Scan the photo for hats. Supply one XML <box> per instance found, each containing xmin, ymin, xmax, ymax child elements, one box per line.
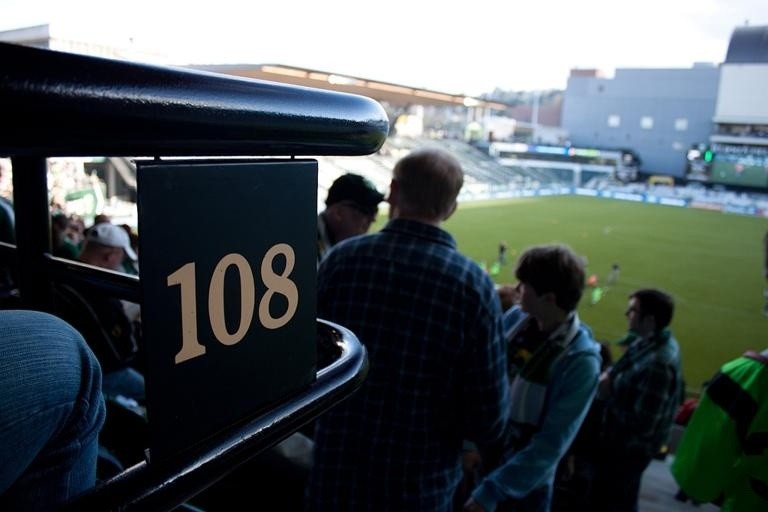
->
<box><xmin>327</xmin><ymin>173</ymin><xmax>383</xmax><ymax>209</ymax></box>
<box><xmin>87</xmin><ymin>223</ymin><xmax>139</xmax><ymax>261</ymax></box>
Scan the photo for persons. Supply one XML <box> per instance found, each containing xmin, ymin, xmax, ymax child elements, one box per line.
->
<box><xmin>316</xmin><ymin>150</ymin><xmax>511</xmax><ymax>512</ymax></box>
<box><xmin>462</xmin><ymin>242</ymin><xmax>681</xmax><ymax>512</ymax></box>
<box><xmin>1</xmin><ymin>310</ymin><xmax>106</xmax><ymax>511</ymax></box>
<box><xmin>669</xmin><ymin>345</ymin><xmax>766</xmax><ymax>511</ymax></box>
<box><xmin>1</xmin><ymin>190</ymin><xmax>146</xmax><ymax>469</ymax></box>
